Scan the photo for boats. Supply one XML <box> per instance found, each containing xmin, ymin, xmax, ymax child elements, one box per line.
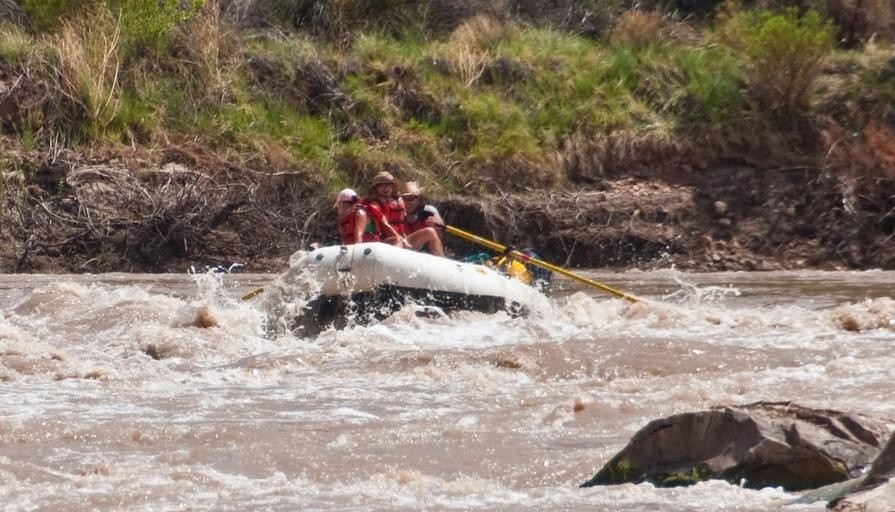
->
<box><xmin>249</xmin><ymin>240</ymin><xmax>555</xmax><ymax>336</ymax></box>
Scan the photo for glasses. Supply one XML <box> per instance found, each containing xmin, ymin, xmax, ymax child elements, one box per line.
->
<box><xmin>379</xmin><ymin>183</ymin><xmax>393</xmax><ymax>187</ymax></box>
<box><xmin>403</xmin><ymin>197</ymin><xmax>414</xmax><ymax>202</ymax></box>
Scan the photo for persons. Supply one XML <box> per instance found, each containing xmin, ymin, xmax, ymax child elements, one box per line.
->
<box><xmin>330</xmin><ymin>187</ymin><xmax>377</xmax><ymax>244</ymax></box>
<box><xmin>397</xmin><ymin>179</ymin><xmax>446</xmax><ymax>255</ymax></box>
<box><xmin>364</xmin><ymin>171</ymin><xmax>446</xmax><ymax>257</ymax></box>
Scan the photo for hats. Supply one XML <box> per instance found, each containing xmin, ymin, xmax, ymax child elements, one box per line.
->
<box><xmin>332</xmin><ymin>188</ymin><xmax>358</xmax><ymax>209</ymax></box>
<box><xmin>368</xmin><ymin>171</ymin><xmax>395</xmax><ymax>189</ymax></box>
<box><xmin>400</xmin><ymin>181</ymin><xmax>420</xmax><ymax>196</ymax></box>
